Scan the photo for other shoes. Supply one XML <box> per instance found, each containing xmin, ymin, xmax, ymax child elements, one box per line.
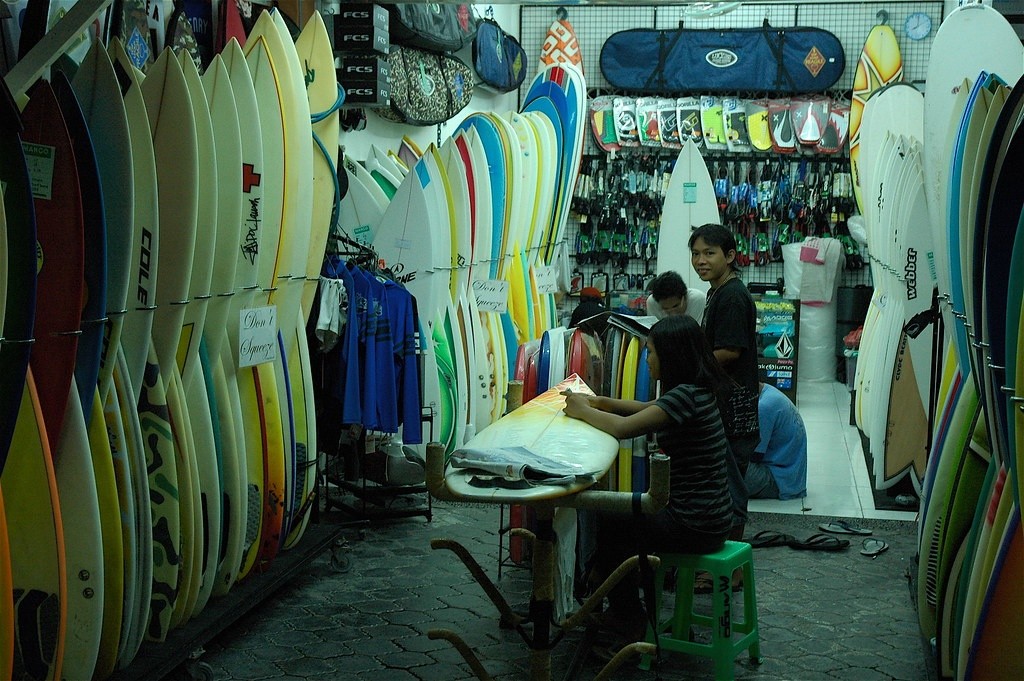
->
<box><xmin>596</xmin><ymin>602</ymin><xmax>647</xmax><ymax>640</ymax></box>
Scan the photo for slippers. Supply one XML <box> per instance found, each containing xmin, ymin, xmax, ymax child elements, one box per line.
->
<box><xmin>741</xmin><ymin>530</ymin><xmax>795</xmax><ymax>547</ymax></box>
<box><xmin>788</xmin><ymin>533</ymin><xmax>849</xmax><ymax>550</ymax></box>
<box><xmin>860</xmin><ymin>538</ymin><xmax>889</xmax><ymax>556</ymax></box>
<box><xmin>819</xmin><ymin>520</ymin><xmax>873</xmax><ymax>536</ymax></box>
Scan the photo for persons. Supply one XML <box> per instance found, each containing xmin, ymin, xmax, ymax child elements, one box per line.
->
<box><xmin>568</xmin><ymin>287</ymin><xmax>608</xmax><ymax>329</ymax></box>
<box><xmin>563</xmin><ymin>316</ymin><xmax>734</xmax><ymax>642</ymax></box>
<box><xmin>646</xmin><ymin>270</ymin><xmax>706</xmax><ymax>326</ymax></box>
<box><xmin>688</xmin><ymin>224</ymin><xmax>758</xmax><ymax>594</ymax></box>
<box><xmin>745</xmin><ymin>382</ymin><xmax>807</xmax><ymax>501</ymax></box>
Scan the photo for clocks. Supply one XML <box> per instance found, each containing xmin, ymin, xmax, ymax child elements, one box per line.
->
<box><xmin>903</xmin><ymin>12</ymin><xmax>932</xmax><ymax>41</ymax></box>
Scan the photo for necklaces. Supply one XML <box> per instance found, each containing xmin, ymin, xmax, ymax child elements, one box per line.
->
<box><xmin>704</xmin><ymin>271</ymin><xmax>734</xmax><ymax>311</ymax></box>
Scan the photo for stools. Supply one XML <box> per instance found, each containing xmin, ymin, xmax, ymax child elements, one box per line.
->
<box><xmin>638</xmin><ymin>541</ymin><xmax>761</xmax><ymax>681</ymax></box>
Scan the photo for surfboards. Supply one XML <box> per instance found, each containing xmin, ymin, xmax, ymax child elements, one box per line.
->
<box><xmin>0</xmin><ymin>4</ymin><xmax>1024</xmax><ymax>680</ymax></box>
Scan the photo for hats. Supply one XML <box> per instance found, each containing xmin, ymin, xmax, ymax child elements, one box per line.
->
<box><xmin>580</xmin><ymin>287</ymin><xmax>602</xmax><ymax>302</ymax></box>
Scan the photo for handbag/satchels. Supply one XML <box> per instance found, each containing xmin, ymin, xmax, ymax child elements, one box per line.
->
<box><xmin>452</xmin><ymin>6</ymin><xmax>527</xmax><ymax>94</ymax></box>
<box><xmin>354</xmin><ymin>44</ymin><xmax>473</xmax><ymax>126</ymax></box>
<box><xmin>599</xmin><ymin>20</ymin><xmax>845</xmax><ymax>89</ymax></box>
<box><xmin>389</xmin><ymin>2</ymin><xmax>478</xmax><ymax>52</ymax></box>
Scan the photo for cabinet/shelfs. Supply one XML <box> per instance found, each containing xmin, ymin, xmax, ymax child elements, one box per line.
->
<box><xmin>325</xmin><ymin>405</ymin><xmax>435</xmax><ymax>523</ymax></box>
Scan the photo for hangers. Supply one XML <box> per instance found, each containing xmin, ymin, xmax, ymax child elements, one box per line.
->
<box><xmin>324</xmin><ymin>238</ymin><xmax>412</xmax><ymax>280</ymax></box>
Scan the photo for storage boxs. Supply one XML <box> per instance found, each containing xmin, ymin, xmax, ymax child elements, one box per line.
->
<box><xmin>333</xmin><ymin>2</ymin><xmax>392</xmax><ymax>106</ymax></box>
